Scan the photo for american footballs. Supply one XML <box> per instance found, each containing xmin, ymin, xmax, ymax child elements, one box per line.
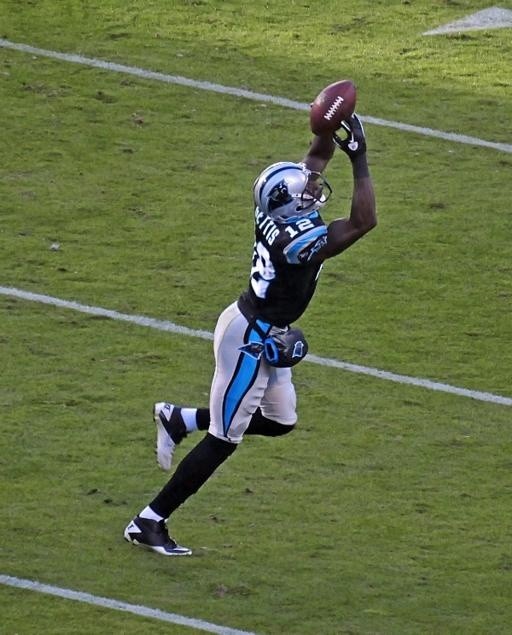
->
<box><xmin>310</xmin><ymin>80</ymin><xmax>356</xmax><ymax>133</ymax></box>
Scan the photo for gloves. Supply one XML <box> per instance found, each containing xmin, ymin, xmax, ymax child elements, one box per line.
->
<box><xmin>329</xmin><ymin>112</ymin><xmax>370</xmax><ymax>164</ymax></box>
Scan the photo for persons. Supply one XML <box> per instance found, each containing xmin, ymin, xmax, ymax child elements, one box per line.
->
<box><xmin>123</xmin><ymin>107</ymin><xmax>380</xmax><ymax>559</ymax></box>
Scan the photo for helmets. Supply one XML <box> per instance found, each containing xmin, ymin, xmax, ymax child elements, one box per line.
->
<box><xmin>250</xmin><ymin>159</ymin><xmax>334</xmax><ymax>224</ymax></box>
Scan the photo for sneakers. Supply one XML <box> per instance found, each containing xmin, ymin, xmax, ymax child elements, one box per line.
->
<box><xmin>121</xmin><ymin>513</ymin><xmax>194</xmax><ymax>558</ymax></box>
<box><xmin>151</xmin><ymin>400</ymin><xmax>192</xmax><ymax>472</ymax></box>
